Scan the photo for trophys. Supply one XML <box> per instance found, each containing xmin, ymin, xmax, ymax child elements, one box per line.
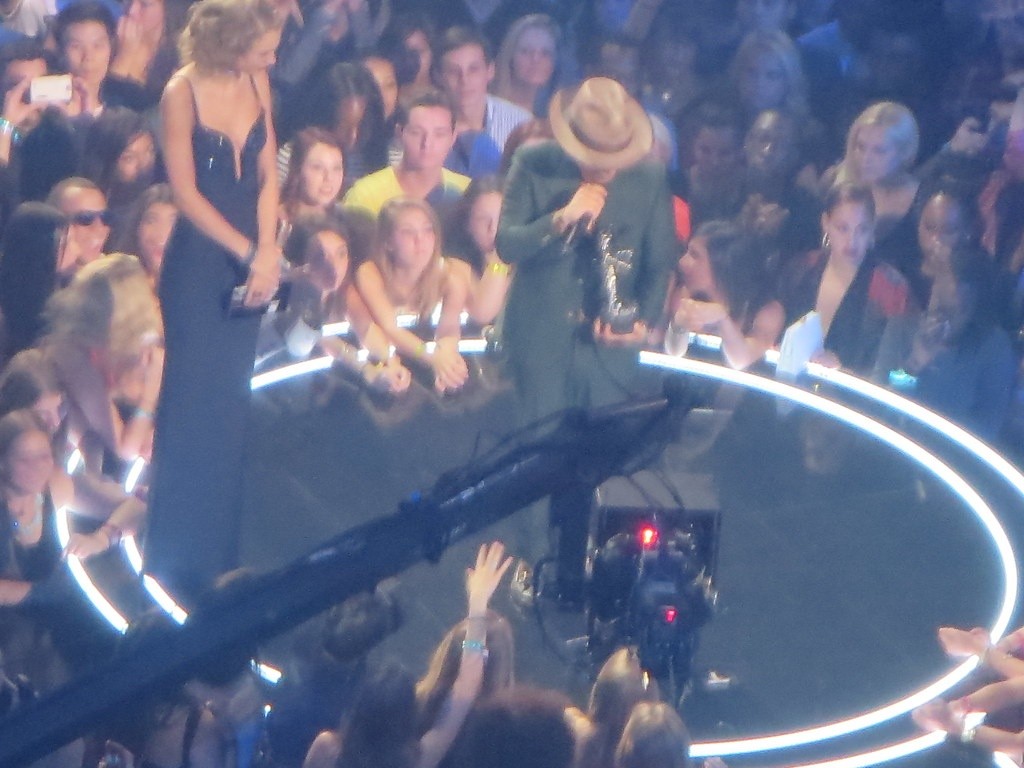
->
<box><xmin>594</xmin><ymin>226</ymin><xmax>640</xmax><ymax>335</ymax></box>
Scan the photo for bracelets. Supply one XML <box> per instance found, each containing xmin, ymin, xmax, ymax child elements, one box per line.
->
<box><xmin>461</xmin><ymin>640</ymin><xmax>483</xmax><ymax>650</ymax></box>
<box><xmin>963</xmin><ymin>697</ymin><xmax>971</xmax><ymax>714</ymax></box>
<box><xmin>12</xmin><ymin>129</ymin><xmax>23</xmax><ymax>147</ymax></box>
<box><xmin>0</xmin><ymin>117</ymin><xmax>13</xmax><ymax>134</ymax></box>
<box><xmin>961</xmin><ymin>727</ymin><xmax>975</xmax><ymax>743</ymax></box>
<box><xmin>978</xmin><ymin>642</ymin><xmax>995</xmax><ymax>664</ymax></box>
<box><xmin>133</xmin><ymin>409</ymin><xmax>157</xmax><ymax>418</ymax></box>
<box><xmin>101</xmin><ymin>524</ymin><xmax>120</xmax><ymax>548</ymax></box>
<box><xmin>487</xmin><ymin>264</ymin><xmax>508</xmax><ymax>274</ymax></box>
<box><xmin>414</xmin><ymin>342</ymin><xmax>427</xmax><ymax>359</ymax></box>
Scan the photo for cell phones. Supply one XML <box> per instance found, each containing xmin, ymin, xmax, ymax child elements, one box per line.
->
<box><xmin>31</xmin><ymin>74</ymin><xmax>73</xmax><ymax>103</ymax></box>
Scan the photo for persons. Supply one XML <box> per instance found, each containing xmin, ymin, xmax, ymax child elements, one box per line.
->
<box><xmin>0</xmin><ymin>0</ymin><xmax>1024</xmax><ymax>768</ymax></box>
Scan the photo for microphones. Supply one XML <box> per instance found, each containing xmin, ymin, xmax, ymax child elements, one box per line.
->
<box><xmin>559</xmin><ymin>222</ymin><xmax>578</xmax><ymax>257</ymax></box>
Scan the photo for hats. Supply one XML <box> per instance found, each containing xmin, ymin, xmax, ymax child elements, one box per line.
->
<box><xmin>550</xmin><ymin>75</ymin><xmax>656</xmax><ymax>171</ymax></box>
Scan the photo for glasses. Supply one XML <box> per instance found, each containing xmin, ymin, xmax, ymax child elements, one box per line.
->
<box><xmin>68</xmin><ymin>209</ymin><xmax>113</xmax><ymax>226</ymax></box>
